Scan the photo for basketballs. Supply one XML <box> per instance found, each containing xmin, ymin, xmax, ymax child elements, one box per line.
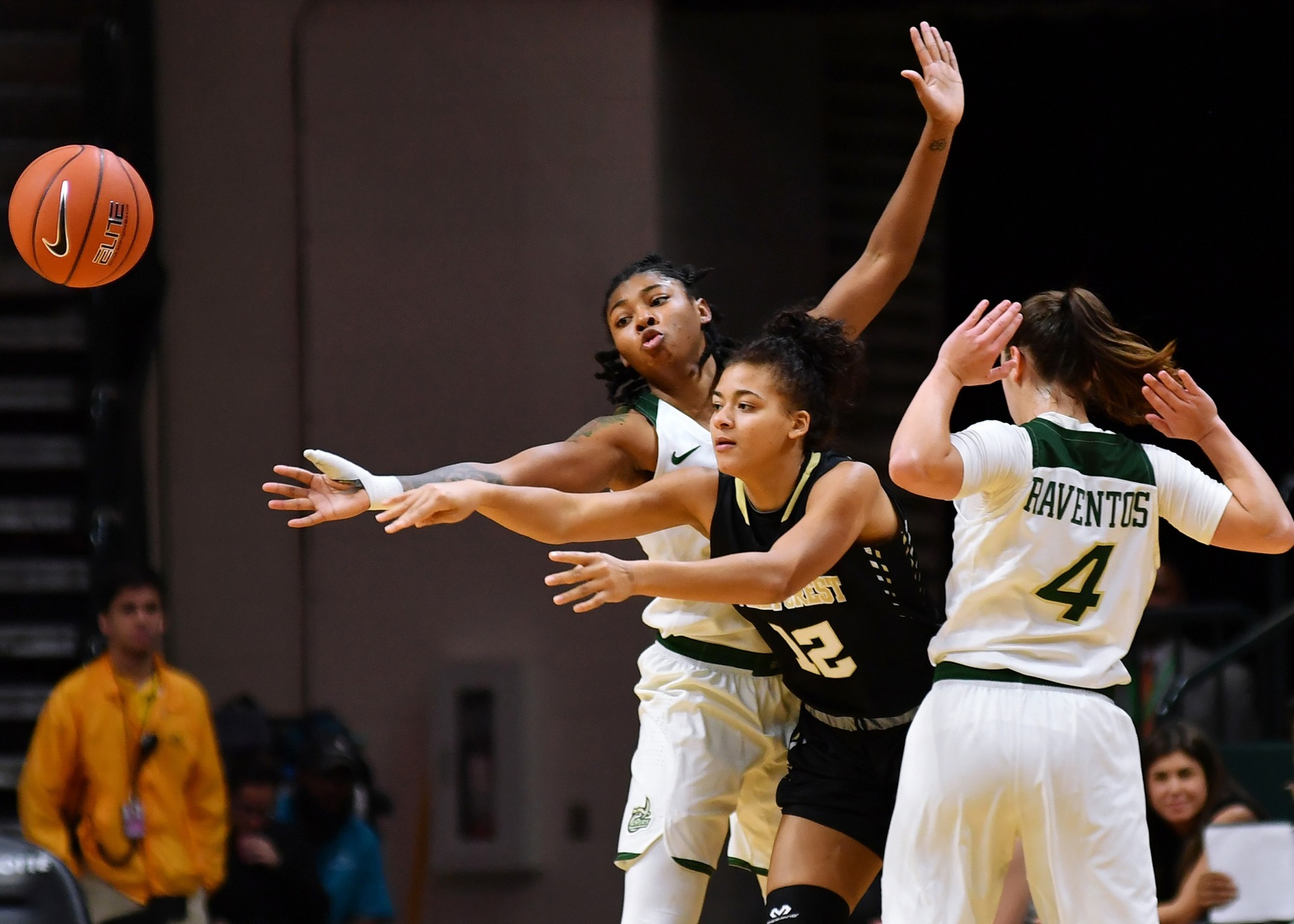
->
<box><xmin>7</xmin><ymin>145</ymin><xmax>154</xmax><ymax>289</ymax></box>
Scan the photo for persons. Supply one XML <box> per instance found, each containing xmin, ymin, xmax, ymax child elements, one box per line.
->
<box><xmin>260</xmin><ymin>19</ymin><xmax>965</xmax><ymax>924</ymax></box>
<box><xmin>18</xmin><ymin>562</ymin><xmax>230</xmax><ymax>924</ymax></box>
<box><xmin>880</xmin><ymin>286</ymin><xmax>1294</xmax><ymax>924</ymax></box>
<box><xmin>373</xmin><ymin>307</ymin><xmax>934</xmax><ymax>924</ymax></box>
<box><xmin>211</xmin><ymin>693</ymin><xmax>391</xmax><ymax>924</ymax></box>
<box><xmin>1145</xmin><ymin>718</ymin><xmax>1253</xmax><ymax>924</ymax></box>
<box><xmin>1119</xmin><ymin>557</ymin><xmax>1255</xmax><ymax>745</ymax></box>
